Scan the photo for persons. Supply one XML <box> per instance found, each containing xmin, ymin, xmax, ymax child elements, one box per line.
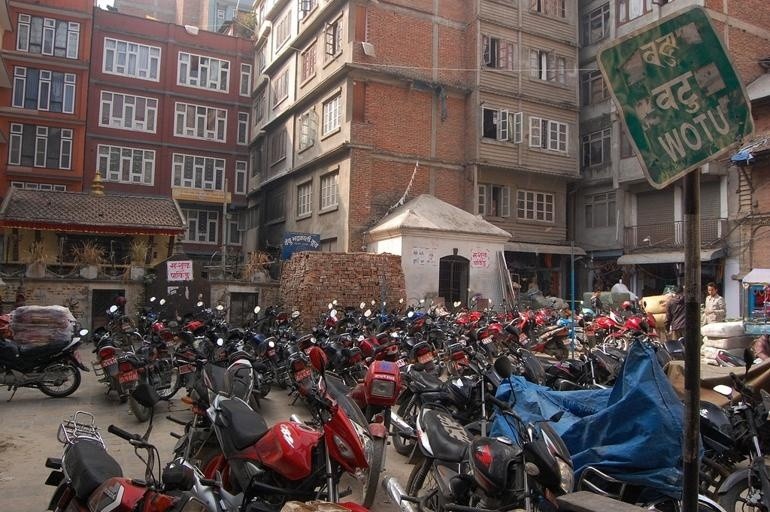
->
<box><xmin>664</xmin><ymin>283</ymin><xmax>686</xmax><ymax>340</ymax></box>
<box><xmin>590</xmin><ymin>288</ymin><xmax>606</xmax><ymax>315</ymax></box>
<box><xmin>751</xmin><ymin>334</ymin><xmax>770</xmax><ymax>360</ymax></box>
<box><xmin>699</xmin><ymin>281</ymin><xmax>726</xmax><ymax>327</ymax></box>
<box><xmin>528</xmin><ymin>276</ymin><xmax>539</xmax><ymax>290</ymax></box>
